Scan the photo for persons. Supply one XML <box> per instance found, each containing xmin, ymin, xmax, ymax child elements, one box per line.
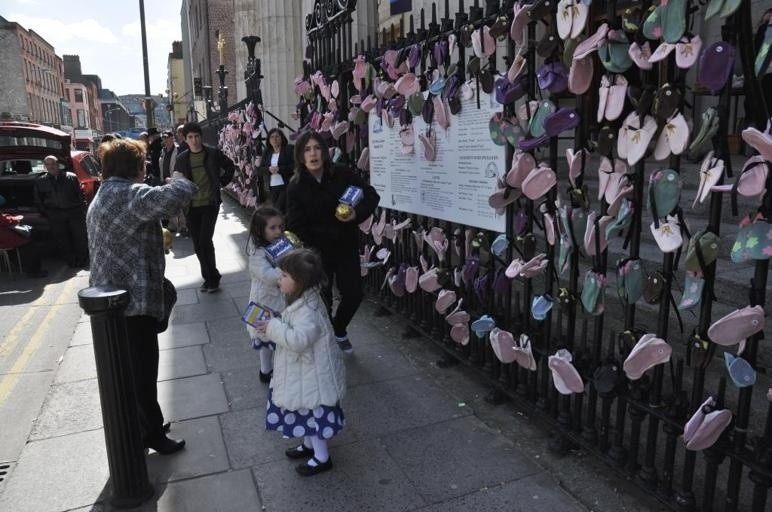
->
<box><xmin>246</xmin><ymin>206</ymin><xmax>284</xmax><ymax>383</ymax></box>
<box><xmin>85</xmin><ymin>137</ymin><xmax>199</xmax><ymax>455</ymax></box>
<box><xmin>33</xmin><ymin>155</ymin><xmax>88</xmax><ymax>269</ymax></box>
<box><xmin>257</xmin><ymin>128</ymin><xmax>296</xmax><ymax>210</ymax></box>
<box><xmin>101</xmin><ymin>121</ymin><xmax>234</xmax><ymax>293</ymax></box>
<box><xmin>1</xmin><ymin>195</ymin><xmax>49</xmax><ymax>278</ymax></box>
<box><xmin>283</xmin><ymin>129</ymin><xmax>381</xmax><ymax>353</ymax></box>
<box><xmin>254</xmin><ymin>247</ymin><xmax>347</xmax><ymax>477</ymax></box>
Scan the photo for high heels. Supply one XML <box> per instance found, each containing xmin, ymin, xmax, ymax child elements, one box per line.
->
<box><xmin>143</xmin><ymin>431</ymin><xmax>185</xmax><ymax>456</ymax></box>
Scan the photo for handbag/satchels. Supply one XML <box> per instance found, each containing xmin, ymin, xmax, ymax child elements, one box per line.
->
<box><xmin>9</xmin><ymin>220</ymin><xmax>33</xmax><ymax>238</ymax></box>
<box><xmin>136</xmin><ymin>273</ymin><xmax>178</xmax><ymax>334</ymax></box>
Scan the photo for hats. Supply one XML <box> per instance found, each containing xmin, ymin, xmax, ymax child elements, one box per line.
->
<box><xmin>162</xmin><ymin>132</ymin><xmax>173</xmax><ymax>138</ymax></box>
<box><xmin>145</xmin><ymin>127</ymin><xmax>160</xmax><ymax>138</ymax></box>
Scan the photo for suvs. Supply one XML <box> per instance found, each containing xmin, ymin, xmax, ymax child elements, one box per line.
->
<box><xmin>0</xmin><ymin>121</ymin><xmax>102</xmax><ymax>260</ymax></box>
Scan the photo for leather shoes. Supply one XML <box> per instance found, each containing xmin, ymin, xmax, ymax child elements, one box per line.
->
<box><xmin>260</xmin><ymin>367</ymin><xmax>272</xmax><ymax>384</ymax></box>
<box><xmin>296</xmin><ymin>455</ymin><xmax>333</xmax><ymax>477</ymax></box>
<box><xmin>285</xmin><ymin>443</ymin><xmax>314</xmax><ymax>458</ymax></box>
<box><xmin>162</xmin><ymin>421</ymin><xmax>171</xmax><ymax>431</ymax></box>
<box><xmin>27</xmin><ymin>269</ymin><xmax>48</xmax><ymax>277</ymax></box>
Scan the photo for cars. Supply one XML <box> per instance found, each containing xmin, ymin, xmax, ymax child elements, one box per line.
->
<box><xmin>117</xmin><ymin>126</ymin><xmax>148</xmax><ymax>140</ymax></box>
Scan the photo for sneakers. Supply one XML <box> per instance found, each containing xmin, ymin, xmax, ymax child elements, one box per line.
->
<box><xmin>207</xmin><ymin>275</ymin><xmax>222</xmax><ymax>293</ymax></box>
<box><xmin>200</xmin><ymin>281</ymin><xmax>208</xmax><ymax>293</ymax></box>
<box><xmin>335</xmin><ymin>334</ymin><xmax>353</xmax><ymax>354</ymax></box>
